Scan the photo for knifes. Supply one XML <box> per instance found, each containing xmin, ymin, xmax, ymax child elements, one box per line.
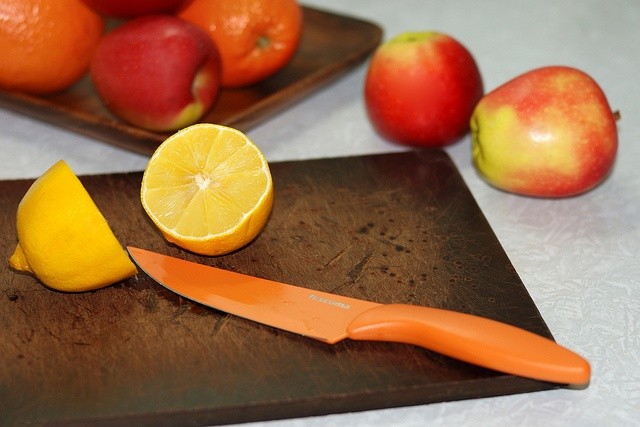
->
<box><xmin>125</xmin><ymin>236</ymin><xmax>593</xmax><ymax>388</ymax></box>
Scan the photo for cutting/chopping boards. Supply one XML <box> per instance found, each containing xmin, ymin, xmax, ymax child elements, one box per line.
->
<box><xmin>0</xmin><ymin>146</ymin><xmax>568</xmax><ymax>427</ymax></box>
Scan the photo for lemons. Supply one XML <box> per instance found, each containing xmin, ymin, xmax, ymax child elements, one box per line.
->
<box><xmin>143</xmin><ymin>122</ymin><xmax>276</xmax><ymax>257</ymax></box>
<box><xmin>11</xmin><ymin>160</ymin><xmax>140</xmax><ymax>292</ymax></box>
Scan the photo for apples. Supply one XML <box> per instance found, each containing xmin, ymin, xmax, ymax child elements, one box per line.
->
<box><xmin>469</xmin><ymin>66</ymin><xmax>618</xmax><ymax>197</ymax></box>
<box><xmin>364</xmin><ymin>32</ymin><xmax>481</xmax><ymax>148</ymax></box>
<box><xmin>94</xmin><ymin>22</ymin><xmax>220</xmax><ymax>129</ymax></box>
